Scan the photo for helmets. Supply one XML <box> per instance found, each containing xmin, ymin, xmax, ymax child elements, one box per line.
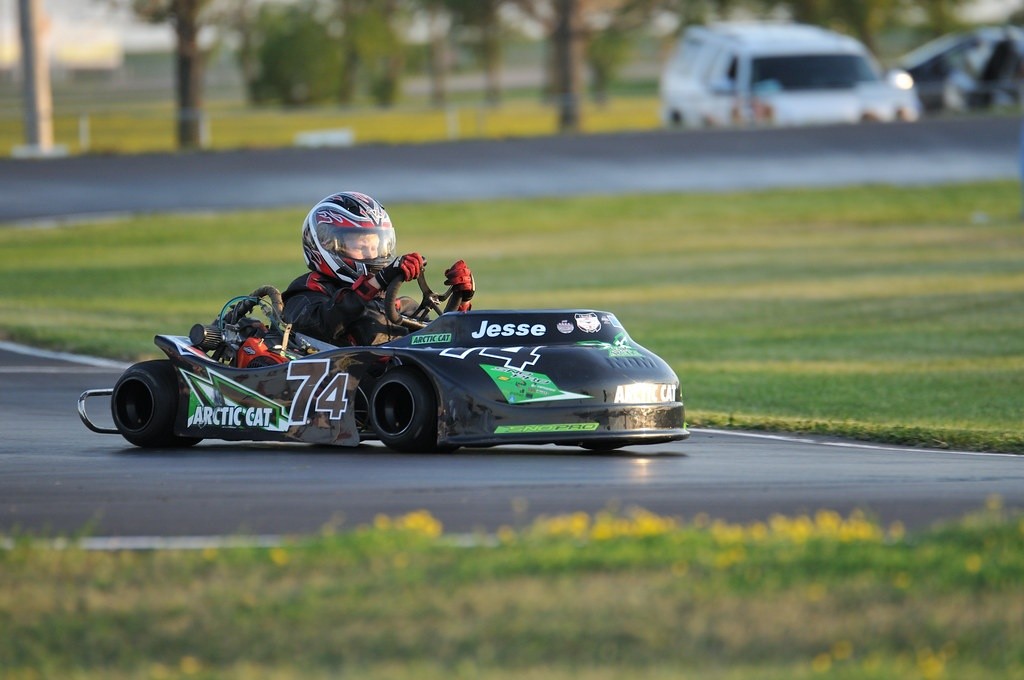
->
<box><xmin>302</xmin><ymin>191</ymin><xmax>396</xmax><ymax>283</ymax></box>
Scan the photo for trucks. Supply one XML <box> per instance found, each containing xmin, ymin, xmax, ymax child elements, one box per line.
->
<box><xmin>657</xmin><ymin>20</ymin><xmax>920</xmax><ymax>128</ymax></box>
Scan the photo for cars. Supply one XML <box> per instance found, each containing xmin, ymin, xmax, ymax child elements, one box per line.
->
<box><xmin>889</xmin><ymin>27</ymin><xmax>1024</xmax><ymax>117</ymax></box>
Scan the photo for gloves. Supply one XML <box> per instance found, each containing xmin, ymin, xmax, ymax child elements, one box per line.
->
<box><xmin>443</xmin><ymin>260</ymin><xmax>476</xmax><ymax>302</ymax></box>
<box><xmin>374</xmin><ymin>253</ymin><xmax>424</xmax><ymax>291</ymax></box>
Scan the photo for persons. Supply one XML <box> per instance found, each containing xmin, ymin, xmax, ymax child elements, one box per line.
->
<box><xmin>282</xmin><ymin>191</ymin><xmax>476</xmax><ymax>348</ymax></box>
